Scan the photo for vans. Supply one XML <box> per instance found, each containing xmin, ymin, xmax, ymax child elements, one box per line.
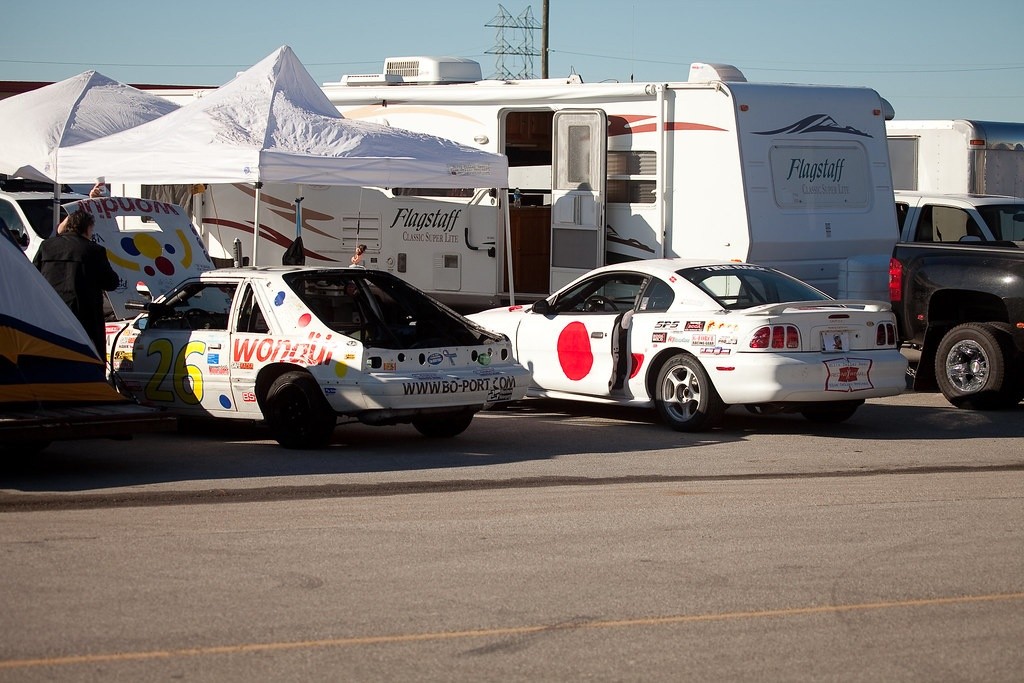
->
<box><xmin>895</xmin><ymin>190</ymin><xmax>1024</xmax><ymax>250</ymax></box>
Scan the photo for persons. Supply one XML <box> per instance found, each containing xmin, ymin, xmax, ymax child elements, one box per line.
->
<box><xmin>32</xmin><ymin>183</ymin><xmax>120</xmax><ymax>365</ymax></box>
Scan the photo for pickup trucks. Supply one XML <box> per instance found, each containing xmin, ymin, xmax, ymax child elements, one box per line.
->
<box><xmin>886</xmin><ymin>238</ymin><xmax>1024</xmax><ymax>414</ymax></box>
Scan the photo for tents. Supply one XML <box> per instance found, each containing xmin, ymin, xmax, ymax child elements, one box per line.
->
<box><xmin>0</xmin><ymin>228</ymin><xmax>141</xmax><ymax>408</ymax></box>
<box><xmin>0</xmin><ymin>68</ymin><xmax>183</xmax><ymax>231</ymax></box>
<box><xmin>53</xmin><ymin>31</ymin><xmax>515</xmax><ymax>307</ymax></box>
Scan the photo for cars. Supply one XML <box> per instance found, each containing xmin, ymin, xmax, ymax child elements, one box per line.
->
<box><xmin>462</xmin><ymin>258</ymin><xmax>911</xmax><ymax>432</ymax></box>
<box><xmin>59</xmin><ymin>198</ymin><xmax>531</xmax><ymax>453</ymax></box>
